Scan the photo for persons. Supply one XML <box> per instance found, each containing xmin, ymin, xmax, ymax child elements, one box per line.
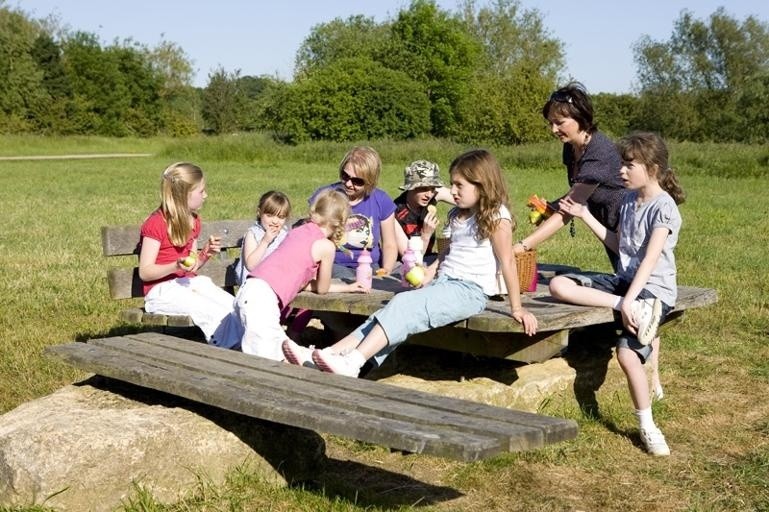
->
<box><xmin>392</xmin><ymin>160</ymin><xmax>457</xmax><ymax>256</ymax></box>
<box><xmin>282</xmin><ymin>150</ymin><xmax>537</xmax><ymax>378</ymax></box>
<box><xmin>234</xmin><ymin>189</ymin><xmax>369</xmax><ymax>361</ymax></box>
<box><xmin>549</xmin><ymin>133</ymin><xmax>686</xmax><ymax>458</ymax></box>
<box><xmin>138</xmin><ymin>162</ymin><xmax>240</xmax><ymax>349</ymax></box>
<box><xmin>235</xmin><ymin>191</ymin><xmax>313</xmax><ymax>340</ymax></box>
<box><xmin>308</xmin><ymin>146</ymin><xmax>398</xmax><ymax>279</ymax></box>
<box><xmin>511</xmin><ymin>80</ymin><xmax>663</xmax><ymax>401</ymax></box>
<box><xmin>342</xmin><ymin>214</ymin><xmax>370</xmax><ymax>248</ymax></box>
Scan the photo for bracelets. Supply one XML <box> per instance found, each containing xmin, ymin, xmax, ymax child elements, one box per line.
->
<box><xmin>519</xmin><ymin>239</ymin><xmax>529</xmax><ymax>251</ymax></box>
<box><xmin>198</xmin><ymin>250</ymin><xmax>210</xmax><ymax>262</ymax></box>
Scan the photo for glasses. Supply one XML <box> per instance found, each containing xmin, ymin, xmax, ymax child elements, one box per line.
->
<box><xmin>341</xmin><ymin>169</ymin><xmax>365</xmax><ymax>187</ymax></box>
<box><xmin>552</xmin><ymin>91</ymin><xmax>575</xmax><ymax>105</ymax></box>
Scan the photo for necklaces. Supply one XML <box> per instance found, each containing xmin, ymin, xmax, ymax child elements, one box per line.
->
<box><xmin>569</xmin><ymin>133</ymin><xmax>591</xmax><ymax>238</ymax></box>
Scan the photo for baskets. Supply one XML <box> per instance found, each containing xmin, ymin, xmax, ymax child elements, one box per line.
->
<box><xmin>436</xmin><ymin>237</ymin><xmax>450</xmax><ymax>253</ymax></box>
<box><xmin>512</xmin><ymin>249</ymin><xmax>537</xmax><ymax>293</ymax></box>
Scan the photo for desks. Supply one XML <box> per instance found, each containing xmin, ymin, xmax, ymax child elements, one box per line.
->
<box><xmin>245</xmin><ymin>265</ymin><xmax>722</xmax><ymax>367</ymax></box>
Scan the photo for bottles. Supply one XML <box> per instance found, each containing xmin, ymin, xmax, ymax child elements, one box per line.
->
<box><xmin>402</xmin><ymin>232</ymin><xmax>426</xmax><ymax>289</ymax></box>
<box><xmin>356</xmin><ymin>247</ymin><xmax>373</xmax><ymax>290</ymax></box>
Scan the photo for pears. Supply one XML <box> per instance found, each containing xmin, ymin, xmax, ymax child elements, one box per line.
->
<box><xmin>184</xmin><ymin>255</ymin><xmax>193</xmax><ymax>268</ymax></box>
<box><xmin>405</xmin><ymin>267</ymin><xmax>424</xmax><ymax>286</ymax></box>
<box><xmin>528</xmin><ymin>210</ymin><xmax>541</xmax><ymax>223</ymax></box>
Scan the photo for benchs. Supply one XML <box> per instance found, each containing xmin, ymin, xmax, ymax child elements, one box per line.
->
<box><xmin>100</xmin><ymin>217</ymin><xmax>305</xmax><ymax>334</ymax></box>
<box><xmin>47</xmin><ymin>332</ymin><xmax>580</xmax><ymax>462</ymax></box>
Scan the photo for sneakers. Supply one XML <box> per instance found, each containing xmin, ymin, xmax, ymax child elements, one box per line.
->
<box><xmin>639</xmin><ymin>427</ymin><xmax>670</xmax><ymax>456</ymax></box>
<box><xmin>282</xmin><ymin>339</ymin><xmax>329</xmax><ymax>366</ymax></box>
<box><xmin>636</xmin><ymin>298</ymin><xmax>662</xmax><ymax>345</ymax></box>
<box><xmin>311</xmin><ymin>344</ymin><xmax>361</xmax><ymax>378</ymax></box>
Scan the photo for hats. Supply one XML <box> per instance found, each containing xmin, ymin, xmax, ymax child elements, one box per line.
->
<box><xmin>399</xmin><ymin>160</ymin><xmax>444</xmax><ymax>190</ymax></box>
